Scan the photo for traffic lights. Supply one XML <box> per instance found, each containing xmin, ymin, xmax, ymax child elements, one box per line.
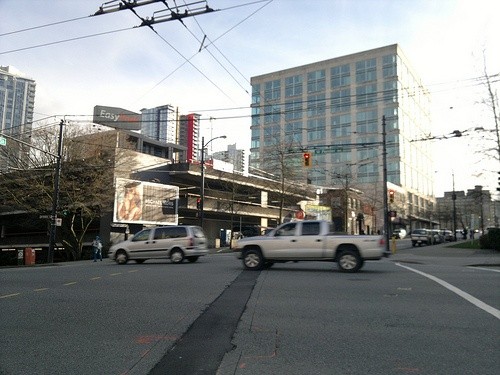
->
<box><xmin>196</xmin><ymin>197</ymin><xmax>202</xmax><ymax>209</ymax></box>
<box><xmin>390</xmin><ymin>190</ymin><xmax>394</xmax><ymax>202</ymax></box>
<box><xmin>304</xmin><ymin>153</ymin><xmax>311</xmax><ymax>167</ymax></box>
<box><xmin>63</xmin><ymin>206</ymin><xmax>69</xmax><ymax>216</ymax></box>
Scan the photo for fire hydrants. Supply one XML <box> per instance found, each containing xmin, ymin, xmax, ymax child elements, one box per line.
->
<box><xmin>391</xmin><ymin>236</ymin><xmax>397</xmax><ymax>255</ymax></box>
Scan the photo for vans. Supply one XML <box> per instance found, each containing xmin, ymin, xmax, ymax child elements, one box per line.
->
<box><xmin>108</xmin><ymin>225</ymin><xmax>208</xmax><ymax>264</ymax></box>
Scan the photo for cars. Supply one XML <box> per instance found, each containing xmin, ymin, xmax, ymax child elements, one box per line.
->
<box><xmin>393</xmin><ymin>229</ymin><xmax>406</xmax><ymax>239</ymax></box>
<box><xmin>412</xmin><ymin>228</ymin><xmax>454</xmax><ymax>246</ymax></box>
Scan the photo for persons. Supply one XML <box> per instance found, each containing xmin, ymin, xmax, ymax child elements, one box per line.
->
<box><xmin>94</xmin><ymin>235</ymin><xmax>103</xmax><ymax>262</ymax></box>
<box><xmin>117</xmin><ymin>182</ymin><xmax>141</xmax><ymax>220</ymax></box>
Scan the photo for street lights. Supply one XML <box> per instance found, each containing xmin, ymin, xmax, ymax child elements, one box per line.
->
<box><xmin>435</xmin><ymin>171</ymin><xmax>456</xmax><ymax>240</ymax></box>
<box><xmin>201</xmin><ymin>136</ymin><xmax>226</xmax><ymax>228</ymax></box>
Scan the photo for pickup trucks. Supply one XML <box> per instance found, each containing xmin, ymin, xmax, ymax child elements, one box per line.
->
<box><xmin>234</xmin><ymin>220</ymin><xmax>386</xmax><ymax>272</ymax></box>
